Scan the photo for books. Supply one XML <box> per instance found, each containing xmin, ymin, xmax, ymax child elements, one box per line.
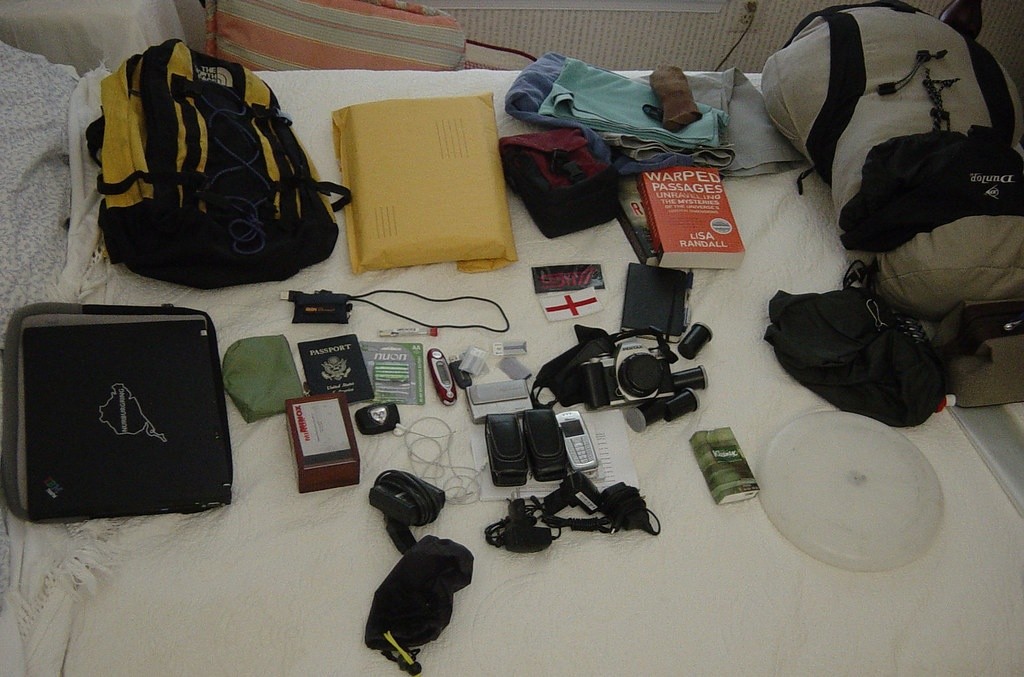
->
<box><xmin>614</xmin><ymin>174</ymin><xmax>659</xmax><ymax>267</ymax></box>
<box><xmin>636</xmin><ymin>165</ymin><xmax>745</xmax><ymax>268</ymax></box>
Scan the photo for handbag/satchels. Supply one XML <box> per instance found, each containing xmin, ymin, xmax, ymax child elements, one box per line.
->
<box><xmin>930</xmin><ymin>298</ymin><xmax>1024</xmax><ymax>407</ymax></box>
<box><xmin>221</xmin><ymin>334</ymin><xmax>304</xmax><ymax>425</ymax></box>
<box><xmin>1</xmin><ymin>303</ymin><xmax>233</xmax><ymax>525</ymax></box>
<box><xmin>763</xmin><ymin>289</ymin><xmax>944</xmax><ymax>428</ymax></box>
<box><xmin>498</xmin><ymin>128</ymin><xmax>620</xmax><ymax>239</ymax></box>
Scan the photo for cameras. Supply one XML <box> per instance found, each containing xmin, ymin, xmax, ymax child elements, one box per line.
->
<box><xmin>576</xmin><ymin>340</ymin><xmax>676</xmax><ymax>411</ymax></box>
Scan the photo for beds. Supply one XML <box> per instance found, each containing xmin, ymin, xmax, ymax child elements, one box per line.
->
<box><xmin>0</xmin><ymin>42</ymin><xmax>1024</xmax><ymax>677</ymax></box>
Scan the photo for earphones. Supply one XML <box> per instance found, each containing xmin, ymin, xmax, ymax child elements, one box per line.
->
<box><xmin>481</xmin><ymin>456</ymin><xmax>489</xmax><ymax>469</ymax></box>
<box><xmin>393</xmin><ymin>423</ymin><xmax>410</xmax><ymax>438</ymax></box>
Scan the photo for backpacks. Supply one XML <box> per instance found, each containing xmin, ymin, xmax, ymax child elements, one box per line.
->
<box><xmin>85</xmin><ymin>38</ymin><xmax>352</xmax><ymax>291</ymax></box>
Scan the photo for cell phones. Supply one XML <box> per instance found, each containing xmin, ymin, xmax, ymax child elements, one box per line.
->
<box><xmin>554</xmin><ymin>410</ymin><xmax>599</xmax><ymax>472</ymax></box>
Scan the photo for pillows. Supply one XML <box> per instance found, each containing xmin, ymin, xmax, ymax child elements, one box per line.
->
<box><xmin>204</xmin><ymin>0</ymin><xmax>466</xmax><ymax>71</ymax></box>
